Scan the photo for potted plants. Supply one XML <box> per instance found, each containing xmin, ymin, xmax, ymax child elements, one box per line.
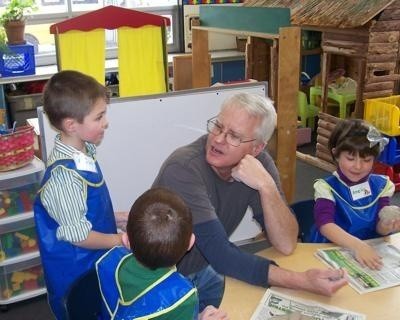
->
<box><xmin>1</xmin><ymin>0</ymin><xmax>36</xmax><ymax>45</ymax></box>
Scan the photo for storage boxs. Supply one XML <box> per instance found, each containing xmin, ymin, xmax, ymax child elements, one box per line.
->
<box><xmin>6</xmin><ymin>91</ymin><xmax>48</xmax><ymax>130</ymax></box>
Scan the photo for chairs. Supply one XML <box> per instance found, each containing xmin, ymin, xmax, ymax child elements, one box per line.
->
<box><xmin>298</xmin><ymin>88</ymin><xmax>323</xmax><ymax>130</ymax></box>
<box><xmin>60</xmin><ymin>267</ymin><xmax>103</xmax><ymax>319</ymax></box>
<box><xmin>290</xmin><ymin>199</ymin><xmax>317</xmax><ymax>244</ymax></box>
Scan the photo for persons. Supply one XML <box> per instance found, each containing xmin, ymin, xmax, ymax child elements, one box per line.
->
<box><xmin>33</xmin><ymin>71</ymin><xmax>122</xmax><ymax>320</ymax></box>
<box><xmin>151</xmin><ymin>92</ymin><xmax>349</xmax><ymax>298</ymax></box>
<box><xmin>95</xmin><ymin>186</ymin><xmax>229</xmax><ymax>320</ymax></box>
<box><xmin>309</xmin><ymin>119</ymin><xmax>400</xmax><ymax>270</ymax></box>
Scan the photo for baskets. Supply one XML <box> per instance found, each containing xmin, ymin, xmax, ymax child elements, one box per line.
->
<box><xmin>0</xmin><ymin>126</ymin><xmax>35</xmax><ymax>172</ymax></box>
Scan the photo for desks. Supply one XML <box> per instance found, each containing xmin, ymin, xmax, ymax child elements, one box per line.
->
<box><xmin>218</xmin><ymin>242</ymin><xmax>400</xmax><ymax>320</ymax></box>
<box><xmin>308</xmin><ymin>85</ymin><xmax>361</xmax><ymax>132</ymax></box>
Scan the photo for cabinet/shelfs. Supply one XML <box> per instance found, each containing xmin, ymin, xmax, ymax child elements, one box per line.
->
<box><xmin>0</xmin><ymin>154</ymin><xmax>48</xmax><ymax>312</ymax></box>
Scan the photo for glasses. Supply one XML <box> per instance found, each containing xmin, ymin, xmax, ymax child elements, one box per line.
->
<box><xmin>207</xmin><ymin>115</ymin><xmax>256</xmax><ymax>146</ymax></box>
